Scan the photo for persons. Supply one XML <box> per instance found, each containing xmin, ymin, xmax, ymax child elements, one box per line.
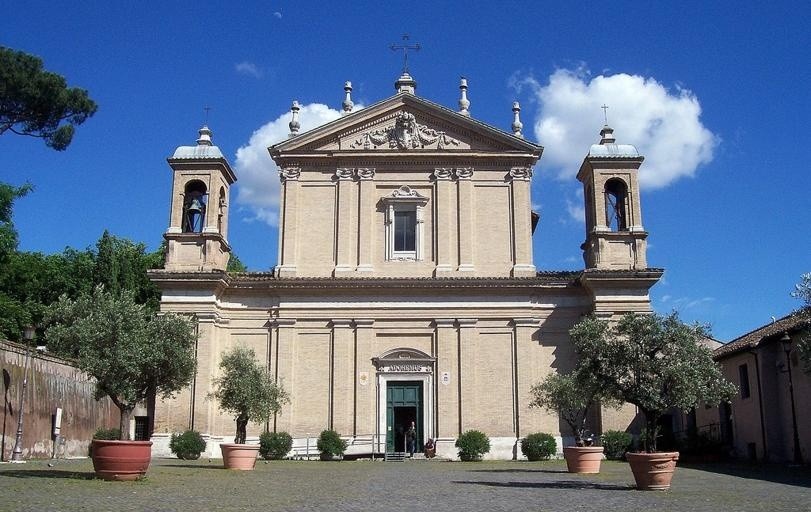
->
<box><xmin>423</xmin><ymin>438</ymin><xmax>436</xmax><ymax>458</ymax></box>
<box><xmin>405</xmin><ymin>427</ymin><xmax>416</xmax><ymax>456</ymax></box>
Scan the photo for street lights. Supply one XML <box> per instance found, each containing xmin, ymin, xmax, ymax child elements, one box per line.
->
<box><xmin>780</xmin><ymin>331</ymin><xmax>808</xmax><ymax>468</ymax></box>
<box><xmin>9</xmin><ymin>319</ymin><xmax>49</xmax><ymax>464</ymax></box>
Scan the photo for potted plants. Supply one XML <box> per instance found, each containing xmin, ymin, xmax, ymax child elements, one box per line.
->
<box><xmin>257</xmin><ymin>431</ymin><xmax>292</xmax><ymax>460</ymax></box>
<box><xmin>568</xmin><ymin>308</ymin><xmax>738</xmax><ymax>490</ymax></box>
<box><xmin>519</xmin><ymin>432</ymin><xmax>557</xmax><ymax>461</ymax></box>
<box><xmin>601</xmin><ymin>430</ymin><xmax>632</xmax><ymax>460</ymax></box>
<box><xmin>170</xmin><ymin>429</ymin><xmax>207</xmax><ymax>459</ymax></box>
<box><xmin>317</xmin><ymin>430</ymin><xmax>350</xmax><ymax>460</ymax></box>
<box><xmin>45</xmin><ymin>283</ymin><xmax>204</xmax><ymax>481</ymax></box>
<box><xmin>454</xmin><ymin>429</ymin><xmax>491</xmax><ymax>461</ymax></box>
<box><xmin>527</xmin><ymin>371</ymin><xmax>622</xmax><ymax>473</ymax></box>
<box><xmin>205</xmin><ymin>341</ymin><xmax>293</xmax><ymax>470</ymax></box>
